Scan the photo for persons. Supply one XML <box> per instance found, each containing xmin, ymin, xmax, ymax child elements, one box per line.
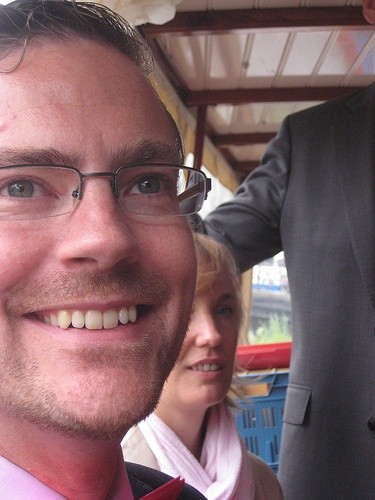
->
<box><xmin>0</xmin><ymin>0</ymin><xmax>209</xmax><ymax>500</ymax></box>
<box><xmin>186</xmin><ymin>0</ymin><xmax>375</xmax><ymax>500</ymax></box>
<box><xmin>120</xmin><ymin>232</ymin><xmax>286</xmax><ymax>500</ymax></box>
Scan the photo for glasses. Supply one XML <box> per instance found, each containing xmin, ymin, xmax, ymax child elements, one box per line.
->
<box><xmin>0</xmin><ymin>162</ymin><xmax>212</xmax><ymax>232</ymax></box>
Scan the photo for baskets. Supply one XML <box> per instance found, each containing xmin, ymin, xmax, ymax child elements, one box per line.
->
<box><xmin>230</xmin><ymin>372</ymin><xmax>286</xmax><ymax>469</ymax></box>
